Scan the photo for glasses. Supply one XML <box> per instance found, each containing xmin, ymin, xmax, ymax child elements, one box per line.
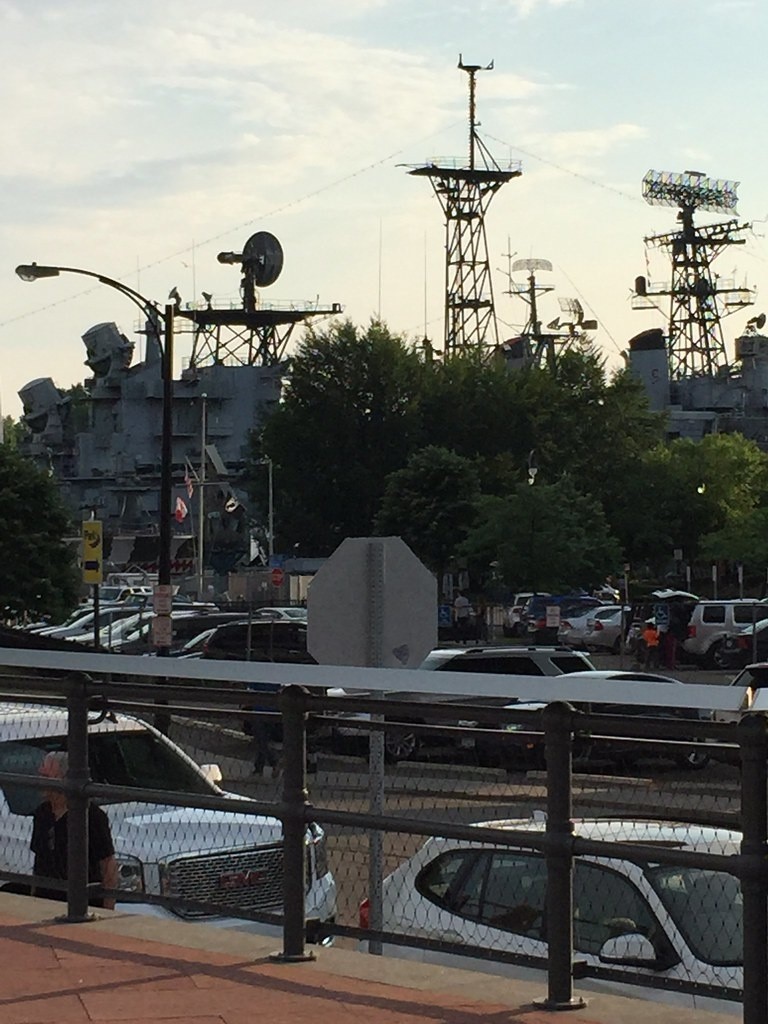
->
<box><xmin>47</xmin><ymin>826</ymin><xmax>55</xmax><ymax>853</ymax></box>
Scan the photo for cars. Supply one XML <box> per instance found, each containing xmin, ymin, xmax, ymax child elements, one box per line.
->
<box><xmin>354</xmin><ymin>814</ymin><xmax>745</xmax><ymax>1020</ymax></box>
<box><xmin>0</xmin><ymin>586</ymin><xmax>768</xmax><ymax>787</ymax></box>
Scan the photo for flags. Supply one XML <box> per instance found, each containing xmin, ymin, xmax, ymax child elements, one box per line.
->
<box><xmin>176</xmin><ymin>498</ymin><xmax>187</xmax><ymax>523</ymax></box>
<box><xmin>185</xmin><ymin>473</ymin><xmax>193</xmax><ymax>498</ymax></box>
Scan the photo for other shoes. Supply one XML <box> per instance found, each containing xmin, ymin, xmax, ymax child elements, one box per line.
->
<box><xmin>252</xmin><ymin>767</ymin><xmax>263</xmax><ymax>776</ymax></box>
<box><xmin>271</xmin><ymin>760</ymin><xmax>280</xmax><ymax>778</ymax></box>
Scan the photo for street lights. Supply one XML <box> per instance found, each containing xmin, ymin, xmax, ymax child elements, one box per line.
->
<box><xmin>14</xmin><ymin>260</ymin><xmax>176</xmax><ymax>659</ymax></box>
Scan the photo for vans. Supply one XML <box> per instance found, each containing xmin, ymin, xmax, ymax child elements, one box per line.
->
<box><xmin>0</xmin><ymin>700</ymin><xmax>341</xmax><ymax>954</ymax></box>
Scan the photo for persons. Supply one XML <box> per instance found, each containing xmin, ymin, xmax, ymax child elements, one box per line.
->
<box><xmin>241</xmin><ymin>702</ymin><xmax>282</xmax><ymax>778</ymax></box>
<box><xmin>30</xmin><ymin>750</ymin><xmax>119</xmax><ymax>909</ymax></box>
<box><xmin>455</xmin><ymin>592</ymin><xmax>470</xmax><ymax>645</ymax></box>
<box><xmin>474</xmin><ymin>595</ymin><xmax>489</xmax><ymax>643</ymax></box>
<box><xmin>638</xmin><ymin>623</ymin><xmax>666</xmax><ymax>670</ymax></box>
